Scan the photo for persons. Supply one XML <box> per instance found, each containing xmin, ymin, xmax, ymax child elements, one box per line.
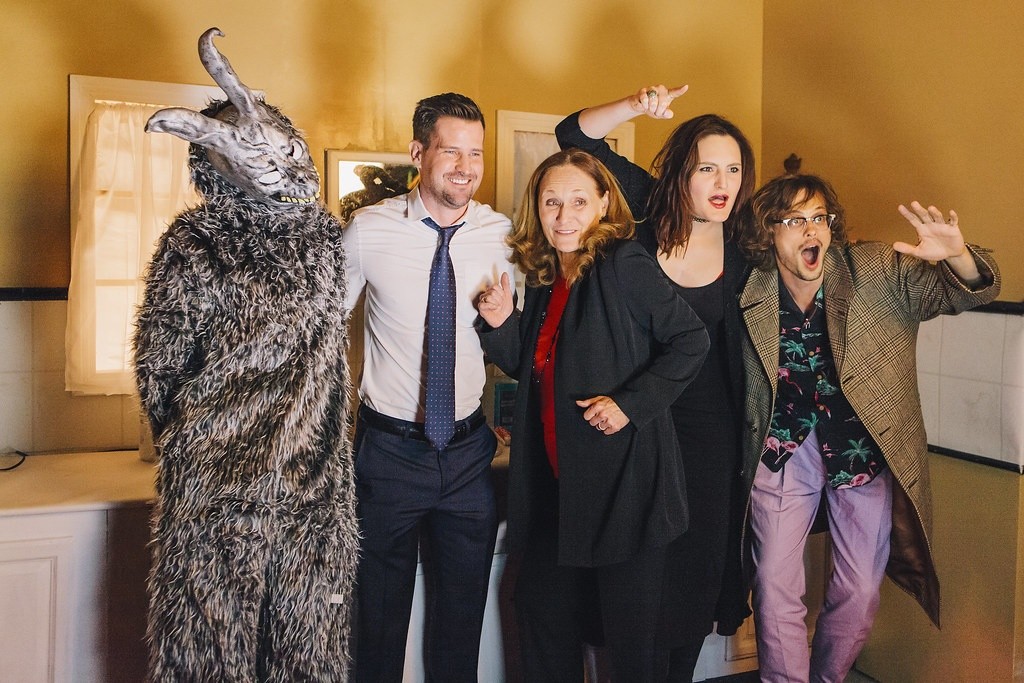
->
<box><xmin>727</xmin><ymin>175</ymin><xmax>1001</xmax><ymax>683</ymax></box>
<box><xmin>477</xmin><ymin>148</ymin><xmax>711</xmax><ymax>683</ymax></box>
<box><xmin>341</xmin><ymin>92</ymin><xmax>515</xmax><ymax>683</ymax></box>
<box><xmin>554</xmin><ymin>84</ymin><xmax>756</xmax><ymax>683</ymax></box>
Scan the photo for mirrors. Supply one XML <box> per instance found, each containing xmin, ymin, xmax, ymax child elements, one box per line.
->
<box><xmin>324</xmin><ymin>149</ymin><xmax>419</xmax><ymax>227</ymax></box>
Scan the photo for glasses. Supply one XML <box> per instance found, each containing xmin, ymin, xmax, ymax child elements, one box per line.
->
<box><xmin>768</xmin><ymin>213</ymin><xmax>836</xmax><ymax>233</ymax></box>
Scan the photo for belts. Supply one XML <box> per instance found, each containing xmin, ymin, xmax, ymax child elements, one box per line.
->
<box><xmin>357</xmin><ymin>404</ymin><xmax>487</xmax><ymax>445</ymax></box>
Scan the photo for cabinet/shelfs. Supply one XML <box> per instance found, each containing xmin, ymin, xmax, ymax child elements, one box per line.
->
<box><xmin>0</xmin><ymin>451</ymin><xmax>823</xmax><ymax>683</ymax></box>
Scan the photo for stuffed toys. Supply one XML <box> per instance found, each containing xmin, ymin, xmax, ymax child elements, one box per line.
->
<box><xmin>133</xmin><ymin>27</ymin><xmax>362</xmax><ymax>683</ymax></box>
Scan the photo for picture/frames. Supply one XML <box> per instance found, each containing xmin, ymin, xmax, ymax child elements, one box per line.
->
<box><xmin>496</xmin><ymin>109</ymin><xmax>636</xmax><ymax>317</ymax></box>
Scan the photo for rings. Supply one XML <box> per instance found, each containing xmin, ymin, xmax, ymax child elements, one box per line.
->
<box><xmin>483</xmin><ymin>297</ymin><xmax>487</xmax><ymax>303</ymax></box>
<box><xmin>598</xmin><ymin>423</ymin><xmax>606</xmax><ymax>430</ymax></box>
<box><xmin>647</xmin><ymin>90</ymin><xmax>656</xmax><ymax>98</ymax></box>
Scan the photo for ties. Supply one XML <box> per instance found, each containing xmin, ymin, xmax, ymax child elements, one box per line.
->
<box><xmin>421</xmin><ymin>217</ymin><xmax>465</xmax><ymax>453</ymax></box>
